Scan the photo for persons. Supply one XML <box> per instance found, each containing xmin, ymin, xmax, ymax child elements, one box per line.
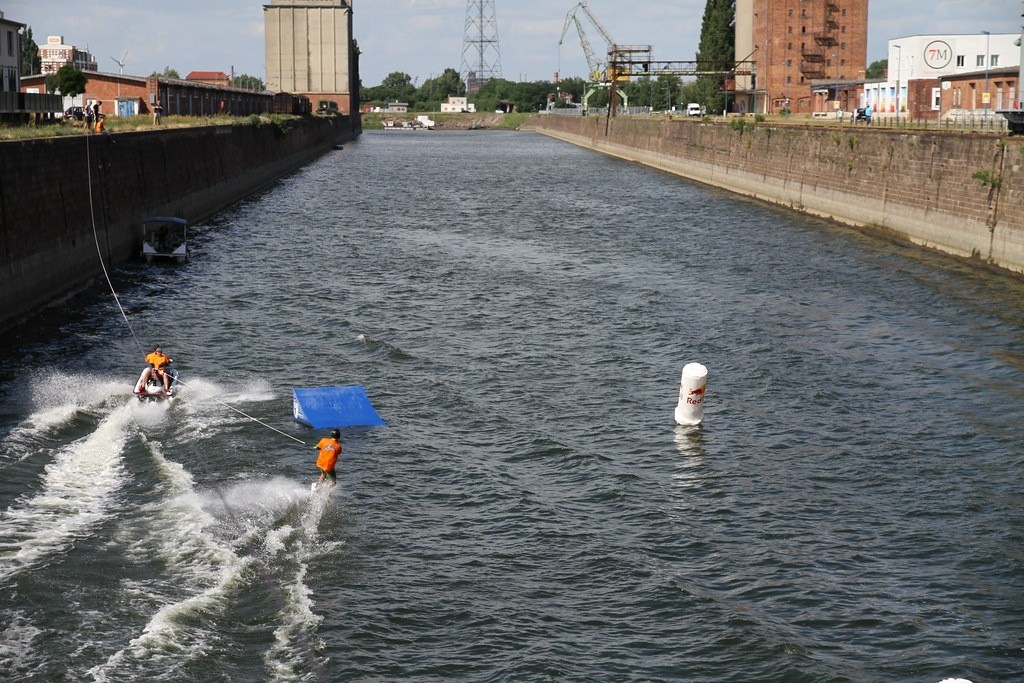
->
<box><xmin>314</xmin><ymin>429</ymin><xmax>342</xmax><ymax>487</ymax></box>
<box><xmin>139</xmin><ymin>347</ymin><xmax>170</xmax><ymax>392</ymax></box>
<box><xmin>153</xmin><ymin>101</ymin><xmax>163</xmax><ymax>125</ymax></box>
<box><xmin>93</xmin><ymin>100</ymin><xmax>101</xmax><ymax>128</ymax></box>
<box><xmin>839</xmin><ymin>110</ymin><xmax>843</xmax><ymax>122</ymax></box>
<box><xmin>83</xmin><ymin>101</ymin><xmax>92</xmax><ymax>131</ymax></box>
<box><xmin>865</xmin><ymin>105</ymin><xmax>872</xmax><ymax>125</ymax></box>
<box><xmin>321</xmin><ymin>104</ymin><xmax>326</xmax><ymax>117</ymax></box>
<box><xmin>96</xmin><ymin>118</ymin><xmax>104</xmax><ymax>133</ymax></box>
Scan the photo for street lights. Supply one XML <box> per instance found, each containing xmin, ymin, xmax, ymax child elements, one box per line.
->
<box><xmin>664</xmin><ymin>81</ymin><xmax>668</xmax><ymax>109</ymax></box>
<box><xmin>893</xmin><ymin>44</ymin><xmax>901</xmax><ymax>115</ymax></box>
<box><xmin>216</xmin><ymin>72</ymin><xmax>222</xmax><ymax>86</ymax></box>
<box><xmin>782</xmin><ymin>62</ymin><xmax>788</xmax><ymax>114</ymax></box>
<box><xmin>661</xmin><ymin>88</ymin><xmax>671</xmax><ymax>110</ymax></box>
<box><xmin>233</xmin><ymin>76</ymin><xmax>239</xmax><ymax>87</ymax></box>
<box><xmin>833</xmin><ymin>54</ymin><xmax>838</xmax><ymax>112</ymax></box>
<box><xmin>983</xmin><ymin>31</ymin><xmax>990</xmax><ymax>121</ymax></box>
<box><xmin>725</xmin><ymin>68</ymin><xmax>734</xmax><ymax>115</ymax></box>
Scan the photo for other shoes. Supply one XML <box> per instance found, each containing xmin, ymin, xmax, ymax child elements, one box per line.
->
<box><xmin>164</xmin><ymin>389</ymin><xmax>172</xmax><ymax>396</ymax></box>
<box><xmin>139</xmin><ymin>385</ymin><xmax>145</xmax><ymax>391</ymax></box>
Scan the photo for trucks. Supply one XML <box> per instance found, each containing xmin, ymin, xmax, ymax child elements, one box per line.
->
<box><xmin>686</xmin><ymin>103</ymin><xmax>701</xmax><ymax>117</ymax></box>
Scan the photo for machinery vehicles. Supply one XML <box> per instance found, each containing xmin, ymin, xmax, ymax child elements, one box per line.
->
<box><xmin>559</xmin><ymin>1</ymin><xmax>630</xmax><ymax>83</ymax></box>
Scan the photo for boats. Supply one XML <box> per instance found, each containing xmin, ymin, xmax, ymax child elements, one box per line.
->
<box><xmin>142</xmin><ymin>216</ymin><xmax>188</xmax><ymax>263</ymax></box>
<box><xmin>133</xmin><ymin>366</ymin><xmax>180</xmax><ymax>403</ymax></box>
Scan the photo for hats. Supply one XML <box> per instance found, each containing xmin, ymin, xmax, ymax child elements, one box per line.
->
<box><xmin>156</xmin><ymin>347</ymin><xmax>161</xmax><ymax>352</ymax></box>
<box><xmin>153</xmin><ymin>344</ymin><xmax>159</xmax><ymax>350</ymax></box>
<box><xmin>330</xmin><ymin>429</ymin><xmax>340</xmax><ymax>439</ymax></box>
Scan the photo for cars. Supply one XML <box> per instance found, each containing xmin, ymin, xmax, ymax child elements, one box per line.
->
<box><xmin>850</xmin><ymin>108</ymin><xmax>871</xmax><ymax>123</ymax></box>
<box><xmin>63</xmin><ymin>107</ymin><xmax>107</xmax><ymax>121</ymax></box>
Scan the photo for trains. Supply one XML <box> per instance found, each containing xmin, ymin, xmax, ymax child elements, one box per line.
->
<box><xmin>316</xmin><ymin>99</ymin><xmax>338</xmax><ymax>115</ymax></box>
<box><xmin>273</xmin><ymin>92</ymin><xmax>312</xmax><ymax>115</ymax></box>
